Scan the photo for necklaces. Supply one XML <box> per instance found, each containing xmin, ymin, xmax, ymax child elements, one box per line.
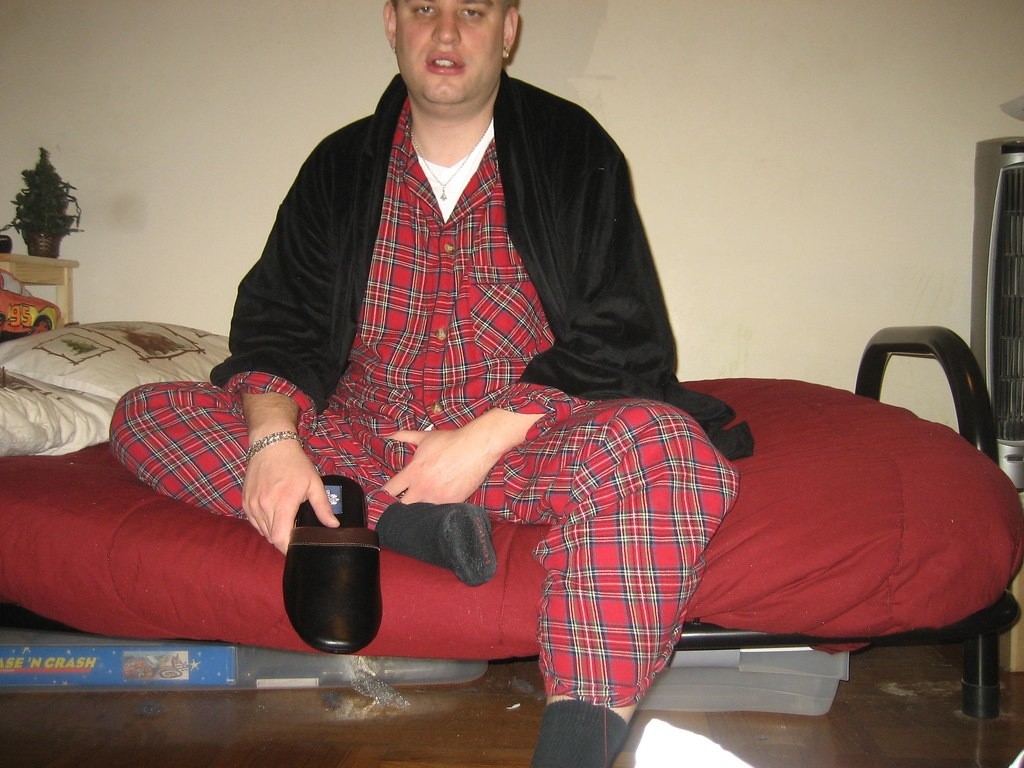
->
<box><xmin>412</xmin><ymin>119</ymin><xmax>491</xmax><ymax>200</ymax></box>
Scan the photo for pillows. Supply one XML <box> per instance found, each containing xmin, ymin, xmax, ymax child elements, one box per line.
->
<box><xmin>0</xmin><ymin>319</ymin><xmax>234</xmax><ymax>451</ymax></box>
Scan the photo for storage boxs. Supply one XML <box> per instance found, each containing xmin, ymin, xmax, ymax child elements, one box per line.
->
<box><xmin>629</xmin><ymin>646</ymin><xmax>849</xmax><ymax>716</ymax></box>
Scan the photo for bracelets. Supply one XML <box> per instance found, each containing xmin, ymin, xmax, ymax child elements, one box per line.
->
<box><xmin>246</xmin><ymin>431</ymin><xmax>304</xmax><ymax>461</ymax></box>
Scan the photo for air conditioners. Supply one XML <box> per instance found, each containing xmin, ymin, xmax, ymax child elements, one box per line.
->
<box><xmin>982</xmin><ymin>136</ymin><xmax>1024</xmax><ymax>490</ymax></box>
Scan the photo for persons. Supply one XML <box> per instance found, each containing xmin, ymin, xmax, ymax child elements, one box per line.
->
<box><xmin>106</xmin><ymin>0</ymin><xmax>753</xmax><ymax>767</ymax></box>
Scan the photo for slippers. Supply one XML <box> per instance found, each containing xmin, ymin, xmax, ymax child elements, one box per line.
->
<box><xmin>281</xmin><ymin>474</ymin><xmax>382</xmax><ymax>653</ymax></box>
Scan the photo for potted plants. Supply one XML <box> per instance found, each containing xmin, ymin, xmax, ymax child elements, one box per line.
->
<box><xmin>0</xmin><ymin>145</ymin><xmax>83</xmax><ymax>258</ymax></box>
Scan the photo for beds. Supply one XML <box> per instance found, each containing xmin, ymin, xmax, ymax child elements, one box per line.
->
<box><xmin>0</xmin><ymin>297</ymin><xmax>1024</xmax><ymax>714</ymax></box>
